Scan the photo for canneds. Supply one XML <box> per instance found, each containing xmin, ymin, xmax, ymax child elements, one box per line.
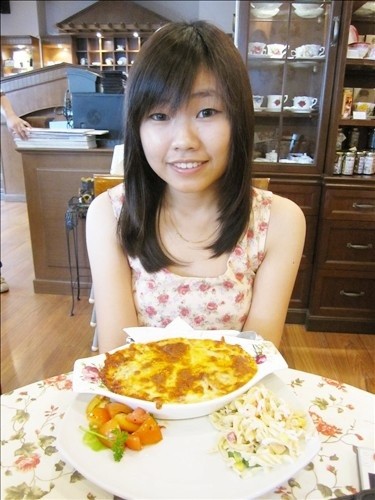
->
<box><xmin>333</xmin><ymin>150</ymin><xmax>375</xmax><ymax>176</ymax></box>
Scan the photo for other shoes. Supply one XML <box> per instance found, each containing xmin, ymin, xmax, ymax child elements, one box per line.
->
<box><xmin>1</xmin><ymin>275</ymin><xmax>9</xmax><ymax>293</ymax></box>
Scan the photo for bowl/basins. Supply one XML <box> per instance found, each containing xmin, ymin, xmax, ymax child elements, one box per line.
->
<box><xmin>250</xmin><ymin>2</ymin><xmax>326</xmax><ymax>19</ymax></box>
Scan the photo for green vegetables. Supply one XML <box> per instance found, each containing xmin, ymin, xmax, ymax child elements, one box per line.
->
<box><xmin>77</xmin><ymin>425</ymin><xmax>128</xmax><ymax>462</ymax></box>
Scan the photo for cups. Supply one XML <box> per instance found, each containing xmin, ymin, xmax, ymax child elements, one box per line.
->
<box><xmin>266</xmin><ymin>94</ymin><xmax>289</xmax><ymax>110</ymax></box>
<box><xmin>251</xmin><ymin>94</ymin><xmax>264</xmax><ymax>110</ymax></box>
<box><xmin>247</xmin><ymin>42</ymin><xmax>267</xmax><ymax>55</ymax></box>
<box><xmin>292</xmin><ymin>96</ymin><xmax>318</xmax><ymax>110</ymax></box>
<box><xmin>290</xmin><ymin>44</ymin><xmax>325</xmax><ymax>57</ymax></box>
<box><xmin>267</xmin><ymin>43</ymin><xmax>295</xmax><ymax>58</ymax></box>
<box><xmin>80</xmin><ymin>58</ymin><xmax>86</xmax><ymax>66</ymax></box>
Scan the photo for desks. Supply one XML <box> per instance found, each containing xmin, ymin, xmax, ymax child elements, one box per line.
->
<box><xmin>0</xmin><ymin>368</ymin><xmax>375</xmax><ymax>500</ymax></box>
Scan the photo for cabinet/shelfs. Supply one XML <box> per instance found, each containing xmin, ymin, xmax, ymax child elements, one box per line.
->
<box><xmin>233</xmin><ymin>0</ymin><xmax>375</xmax><ymax>336</ymax></box>
<box><xmin>70</xmin><ymin>34</ymin><xmax>151</xmax><ymax>76</ymax></box>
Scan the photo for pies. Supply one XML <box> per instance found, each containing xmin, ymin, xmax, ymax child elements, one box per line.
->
<box><xmin>99</xmin><ymin>338</ymin><xmax>258</xmax><ymax>404</ymax></box>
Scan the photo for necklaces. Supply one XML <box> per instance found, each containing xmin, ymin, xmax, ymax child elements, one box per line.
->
<box><xmin>165</xmin><ymin>201</ymin><xmax>220</xmax><ymax>243</ymax></box>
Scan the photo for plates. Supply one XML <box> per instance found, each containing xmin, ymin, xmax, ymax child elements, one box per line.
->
<box><xmin>250</xmin><ymin>106</ymin><xmax>318</xmax><ymax>114</ymax></box>
<box><xmin>82</xmin><ymin>334</ymin><xmax>277</xmax><ymax>421</ymax></box>
<box><xmin>56</xmin><ymin>390</ymin><xmax>321</xmax><ymax>499</ymax></box>
<box><xmin>118</xmin><ymin>56</ymin><xmax>126</xmax><ymax>65</ymax></box>
<box><xmin>246</xmin><ymin>54</ymin><xmax>324</xmax><ymax>67</ymax></box>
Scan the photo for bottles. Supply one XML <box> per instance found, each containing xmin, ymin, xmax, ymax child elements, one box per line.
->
<box><xmin>334</xmin><ymin>126</ymin><xmax>375</xmax><ymax>175</ymax></box>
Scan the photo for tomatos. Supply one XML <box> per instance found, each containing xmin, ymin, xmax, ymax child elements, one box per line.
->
<box><xmin>93</xmin><ymin>402</ymin><xmax>164</xmax><ymax>451</ymax></box>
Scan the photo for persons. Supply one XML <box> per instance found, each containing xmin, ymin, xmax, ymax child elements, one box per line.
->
<box><xmin>86</xmin><ymin>21</ymin><xmax>305</xmax><ymax>355</ymax></box>
<box><xmin>0</xmin><ymin>89</ymin><xmax>31</xmax><ymax>294</ymax></box>
